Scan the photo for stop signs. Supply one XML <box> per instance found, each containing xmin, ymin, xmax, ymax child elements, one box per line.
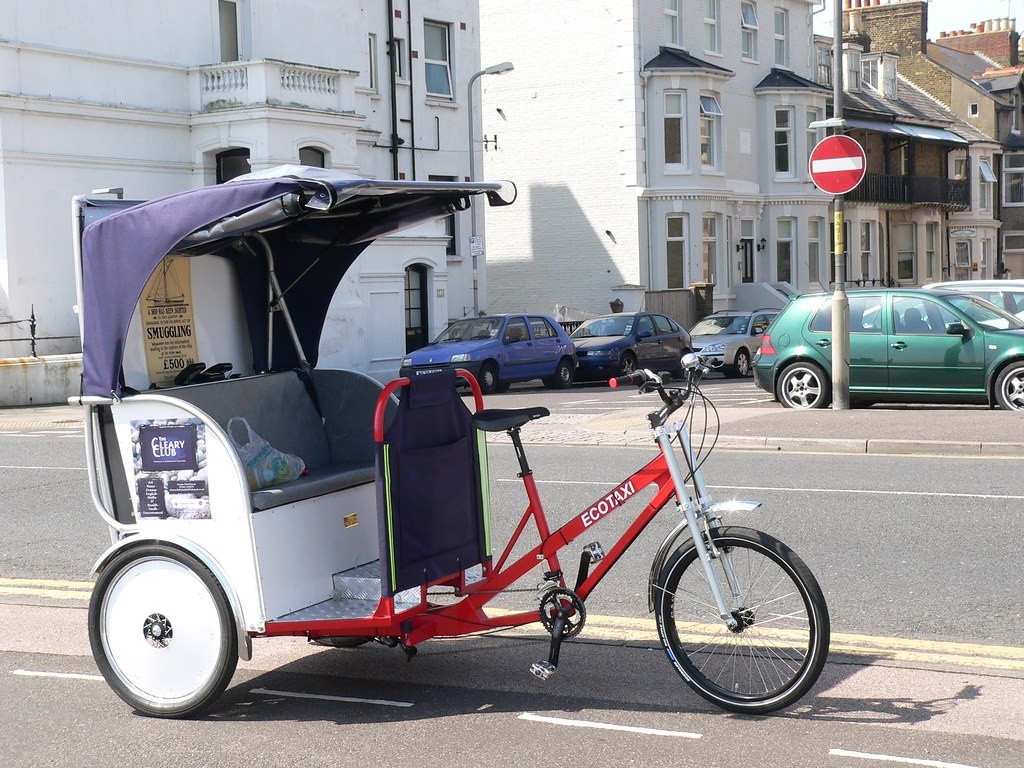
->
<box><xmin>808</xmin><ymin>135</ymin><xmax>866</xmax><ymax>195</ymax></box>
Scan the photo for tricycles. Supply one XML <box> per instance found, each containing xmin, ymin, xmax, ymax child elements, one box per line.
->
<box><xmin>67</xmin><ymin>165</ymin><xmax>830</xmax><ymax>718</ymax></box>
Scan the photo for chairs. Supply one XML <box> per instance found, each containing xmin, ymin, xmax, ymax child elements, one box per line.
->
<box><xmin>893</xmin><ymin>308</ymin><xmax>931</xmax><ymax>334</ymax></box>
<box><xmin>478</xmin><ymin>330</ymin><xmax>490</xmax><ymax>337</ymax></box>
<box><xmin>638</xmin><ymin>322</ymin><xmax>651</xmax><ymax>333</ymax></box>
<box><xmin>508</xmin><ymin>328</ymin><xmax>523</xmax><ymax>338</ymax></box>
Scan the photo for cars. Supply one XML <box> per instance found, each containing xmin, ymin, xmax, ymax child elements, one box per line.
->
<box><xmin>399</xmin><ymin>314</ymin><xmax>578</xmax><ymax>394</ymax></box>
<box><xmin>569</xmin><ymin>312</ymin><xmax>694</xmax><ymax>385</ymax></box>
<box><xmin>751</xmin><ymin>288</ymin><xmax>1024</xmax><ymax>410</ymax></box>
<box><xmin>862</xmin><ymin>279</ymin><xmax>1024</xmax><ymax>330</ymax></box>
<box><xmin>689</xmin><ymin>308</ymin><xmax>781</xmax><ymax>378</ymax></box>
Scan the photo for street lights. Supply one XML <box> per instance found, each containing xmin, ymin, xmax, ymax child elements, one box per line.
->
<box><xmin>468</xmin><ymin>62</ymin><xmax>514</xmax><ymax>317</ymax></box>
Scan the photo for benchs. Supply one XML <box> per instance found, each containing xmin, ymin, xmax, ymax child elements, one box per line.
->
<box><xmin>97</xmin><ymin>369</ymin><xmax>403</xmax><ymax>525</ymax></box>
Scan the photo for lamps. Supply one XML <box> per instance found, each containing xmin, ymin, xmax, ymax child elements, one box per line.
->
<box><xmin>758</xmin><ymin>237</ymin><xmax>766</xmax><ymax>252</ymax></box>
<box><xmin>737</xmin><ymin>237</ymin><xmax>745</xmax><ymax>251</ymax></box>
